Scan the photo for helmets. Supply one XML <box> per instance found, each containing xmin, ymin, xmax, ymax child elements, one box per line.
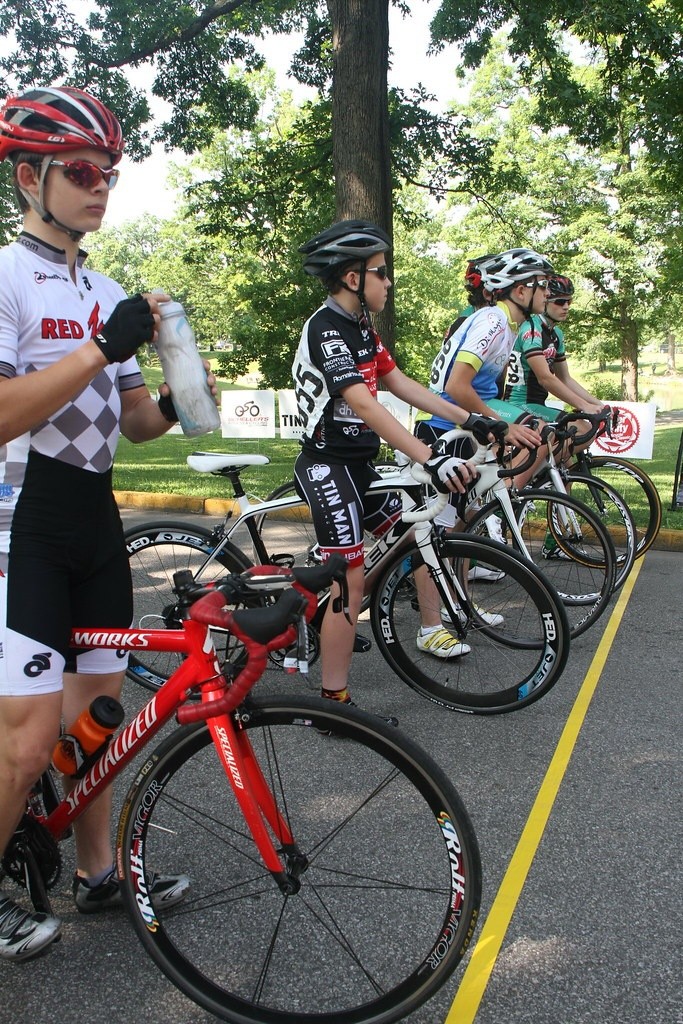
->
<box><xmin>547</xmin><ymin>274</ymin><xmax>575</xmax><ymax>296</ymax></box>
<box><xmin>464</xmin><ymin>253</ymin><xmax>499</xmax><ymax>293</ymax></box>
<box><xmin>469</xmin><ymin>247</ymin><xmax>556</xmax><ymax>293</ymax></box>
<box><xmin>0</xmin><ymin>86</ymin><xmax>124</xmax><ymax>161</ymax></box>
<box><xmin>296</xmin><ymin>219</ymin><xmax>393</xmax><ymax>279</ymax></box>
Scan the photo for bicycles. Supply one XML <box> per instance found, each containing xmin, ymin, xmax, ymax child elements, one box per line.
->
<box><xmin>253</xmin><ymin>404</ymin><xmax>662</xmax><ymax>652</ymax></box>
<box><xmin>1</xmin><ymin>552</ymin><xmax>483</xmax><ymax>1024</ymax></box>
<box><xmin>124</xmin><ymin>419</ymin><xmax>571</xmax><ymax>716</ymax></box>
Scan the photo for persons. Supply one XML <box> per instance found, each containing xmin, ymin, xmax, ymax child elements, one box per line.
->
<box><xmin>0</xmin><ymin>86</ymin><xmax>222</xmax><ymax>965</ymax></box>
<box><xmin>414</xmin><ymin>248</ymin><xmax>604</xmax><ymax>657</ymax></box>
<box><xmin>294</xmin><ymin>219</ymin><xmax>497</xmax><ymax>736</ymax></box>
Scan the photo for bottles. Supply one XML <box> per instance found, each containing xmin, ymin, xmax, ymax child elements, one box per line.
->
<box><xmin>53</xmin><ymin>695</ymin><xmax>126</xmax><ymax>774</ymax></box>
<box><xmin>149</xmin><ymin>286</ymin><xmax>222</xmax><ymax>438</ymax></box>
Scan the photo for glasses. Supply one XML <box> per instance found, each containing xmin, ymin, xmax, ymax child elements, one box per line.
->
<box><xmin>546</xmin><ymin>298</ymin><xmax>573</xmax><ymax>306</ymax></box>
<box><xmin>32</xmin><ymin>159</ymin><xmax>121</xmax><ymax>191</ymax></box>
<box><xmin>337</xmin><ymin>266</ymin><xmax>390</xmax><ymax>280</ymax></box>
<box><xmin>513</xmin><ymin>280</ymin><xmax>549</xmax><ymax>290</ymax></box>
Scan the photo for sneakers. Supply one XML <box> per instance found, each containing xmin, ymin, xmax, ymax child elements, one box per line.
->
<box><xmin>311</xmin><ymin>699</ymin><xmax>399</xmax><ymax>738</ymax></box>
<box><xmin>467</xmin><ymin>563</ymin><xmax>507</xmax><ymax>583</ymax></box>
<box><xmin>542</xmin><ymin>544</ymin><xmax>589</xmax><ymax>562</ymax></box>
<box><xmin>443</xmin><ymin>603</ymin><xmax>504</xmax><ymax>629</ymax></box>
<box><xmin>0</xmin><ymin>889</ymin><xmax>70</xmax><ymax>960</ymax></box>
<box><xmin>73</xmin><ymin>860</ymin><xmax>193</xmax><ymax>914</ymax></box>
<box><xmin>416</xmin><ymin>628</ymin><xmax>471</xmax><ymax>659</ymax></box>
<box><xmin>485</xmin><ymin>510</ymin><xmax>509</xmax><ymax>545</ymax></box>
